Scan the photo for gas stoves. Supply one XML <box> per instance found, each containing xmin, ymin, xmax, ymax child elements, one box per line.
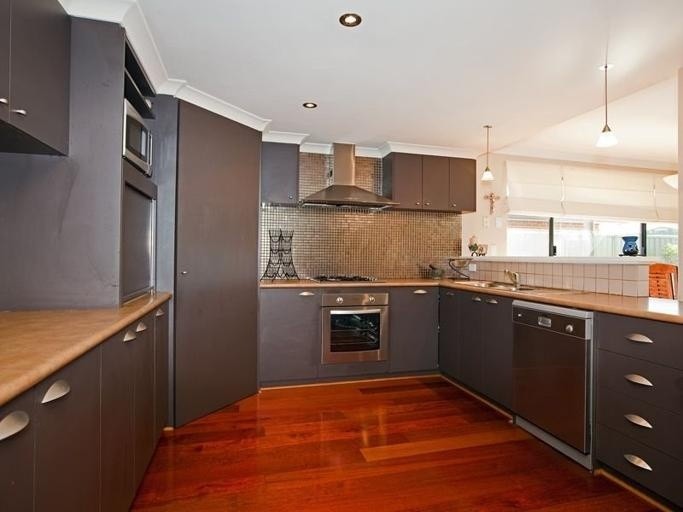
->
<box><xmin>309</xmin><ymin>275</ymin><xmax>385</xmax><ymax>283</ymax></box>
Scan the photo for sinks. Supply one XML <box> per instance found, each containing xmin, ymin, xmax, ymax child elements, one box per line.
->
<box><xmin>475</xmin><ymin>282</ymin><xmax>534</xmax><ymax>292</ymax></box>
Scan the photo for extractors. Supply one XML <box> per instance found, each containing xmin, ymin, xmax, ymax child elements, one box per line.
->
<box><xmin>301</xmin><ymin>143</ymin><xmax>400</xmax><ymax>210</ymax></box>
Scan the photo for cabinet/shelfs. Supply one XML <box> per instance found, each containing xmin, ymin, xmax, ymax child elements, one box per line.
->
<box><xmin>438</xmin><ymin>287</ymin><xmax>514</xmax><ymax>413</ymax></box>
<box><xmin>260</xmin><ymin>141</ymin><xmax>299</xmax><ymax>208</ymax></box>
<box><xmin>382</xmin><ymin>152</ymin><xmax>476</xmax><ymax>214</ymax></box>
<box><xmin>0</xmin><ymin>0</ymin><xmax>72</xmax><ymax>156</ymax></box>
<box><xmin>256</xmin><ymin>288</ymin><xmax>322</xmax><ymax>385</ymax></box>
<box><xmin>591</xmin><ymin>309</ymin><xmax>683</xmax><ymax>511</ymax></box>
<box><xmin>0</xmin><ymin>301</ymin><xmax>169</xmax><ymax>512</ymax></box>
<box><xmin>389</xmin><ymin>286</ymin><xmax>439</xmax><ymax>373</ymax></box>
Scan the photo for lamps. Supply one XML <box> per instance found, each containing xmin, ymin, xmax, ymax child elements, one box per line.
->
<box><xmin>481</xmin><ymin>125</ymin><xmax>495</xmax><ymax>182</ymax></box>
<box><xmin>595</xmin><ymin>62</ymin><xmax>621</xmax><ymax>148</ymax></box>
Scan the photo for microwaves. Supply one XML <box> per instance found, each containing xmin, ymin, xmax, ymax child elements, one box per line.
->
<box><xmin>121</xmin><ymin>95</ymin><xmax>154</xmax><ymax>179</ymax></box>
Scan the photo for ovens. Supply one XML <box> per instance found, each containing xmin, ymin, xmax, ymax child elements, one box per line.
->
<box><xmin>320</xmin><ymin>292</ymin><xmax>390</xmax><ymax>365</ymax></box>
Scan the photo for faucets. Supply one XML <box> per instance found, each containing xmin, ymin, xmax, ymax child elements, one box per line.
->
<box><xmin>504</xmin><ymin>268</ymin><xmax>519</xmax><ymax>288</ymax></box>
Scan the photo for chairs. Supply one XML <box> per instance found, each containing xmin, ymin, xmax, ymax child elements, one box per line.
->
<box><xmin>649</xmin><ymin>263</ymin><xmax>678</xmax><ymax>299</ymax></box>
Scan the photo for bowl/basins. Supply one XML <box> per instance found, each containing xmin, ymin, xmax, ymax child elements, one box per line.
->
<box><xmin>447</xmin><ymin>258</ymin><xmax>472</xmax><ymax>268</ymax></box>
